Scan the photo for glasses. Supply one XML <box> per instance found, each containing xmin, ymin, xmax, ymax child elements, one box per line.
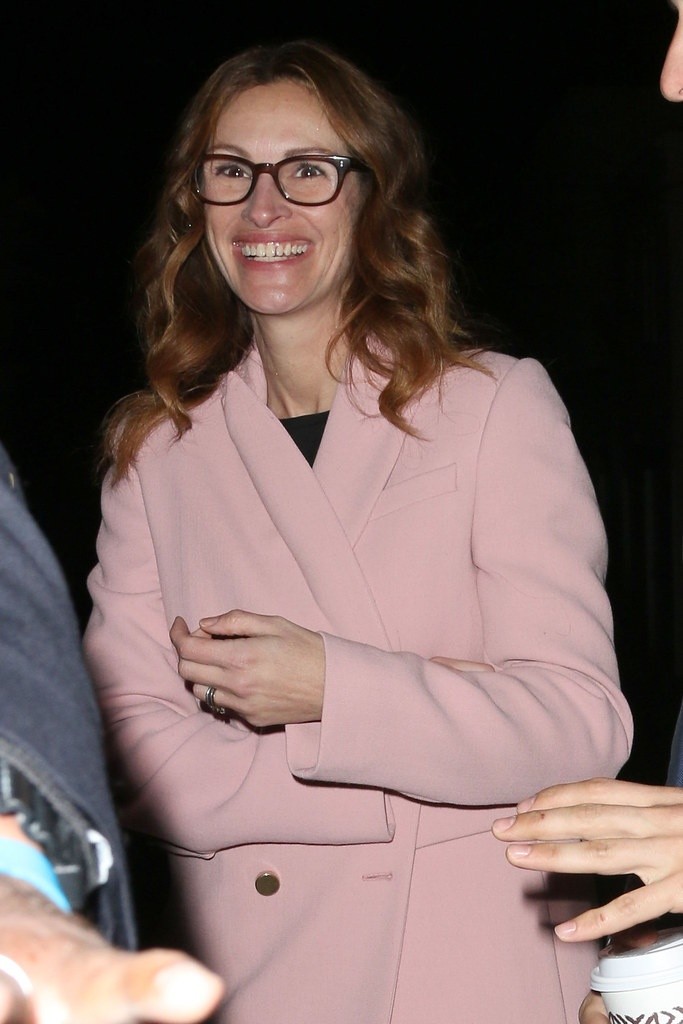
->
<box><xmin>188</xmin><ymin>153</ymin><xmax>357</xmax><ymax>207</ymax></box>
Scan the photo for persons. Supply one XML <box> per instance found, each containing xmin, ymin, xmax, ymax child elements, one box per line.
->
<box><xmin>0</xmin><ymin>457</ymin><xmax>226</xmax><ymax>1024</ymax></box>
<box><xmin>492</xmin><ymin>0</ymin><xmax>683</xmax><ymax>1024</ymax></box>
<box><xmin>85</xmin><ymin>37</ymin><xmax>637</xmax><ymax>1024</ymax></box>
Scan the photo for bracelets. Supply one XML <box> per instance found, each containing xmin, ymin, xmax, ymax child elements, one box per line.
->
<box><xmin>0</xmin><ymin>838</ymin><xmax>73</xmax><ymax>912</ymax></box>
<box><xmin>0</xmin><ymin>764</ymin><xmax>85</xmax><ymax>908</ymax></box>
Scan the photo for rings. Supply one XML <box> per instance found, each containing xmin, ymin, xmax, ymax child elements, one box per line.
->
<box><xmin>205</xmin><ymin>686</ymin><xmax>224</xmax><ymax>715</ymax></box>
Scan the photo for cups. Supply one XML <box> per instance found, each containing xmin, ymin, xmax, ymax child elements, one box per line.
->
<box><xmin>588</xmin><ymin>927</ymin><xmax>683</xmax><ymax>1024</ymax></box>
